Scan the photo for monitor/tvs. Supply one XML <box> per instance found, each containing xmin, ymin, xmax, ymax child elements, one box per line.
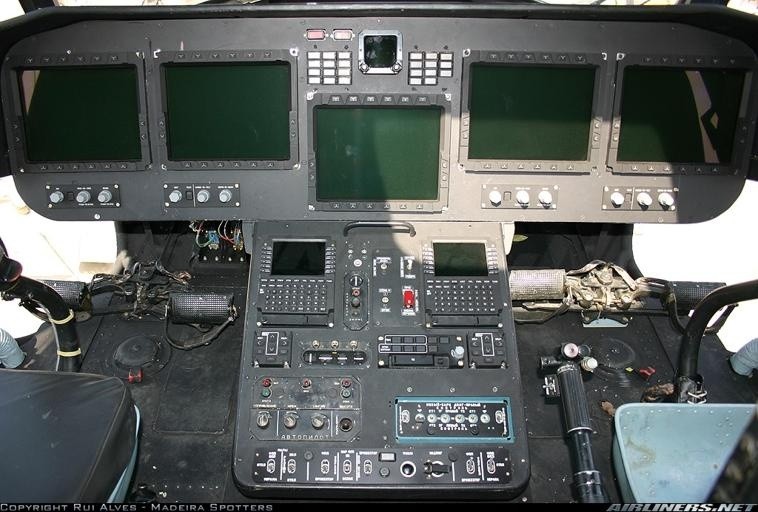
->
<box><xmin>306</xmin><ymin>90</ymin><xmax>453</xmax><ymax>213</ymax></box>
<box><xmin>149</xmin><ymin>47</ymin><xmax>299</xmax><ymax>171</ymax></box>
<box><xmin>457</xmin><ymin>49</ymin><xmax>611</xmax><ymax>175</ymax></box>
<box><xmin>2</xmin><ymin>51</ymin><xmax>151</xmax><ymax>174</ymax></box>
<box><xmin>603</xmin><ymin>52</ymin><xmax>747</xmax><ymax>173</ymax></box>
